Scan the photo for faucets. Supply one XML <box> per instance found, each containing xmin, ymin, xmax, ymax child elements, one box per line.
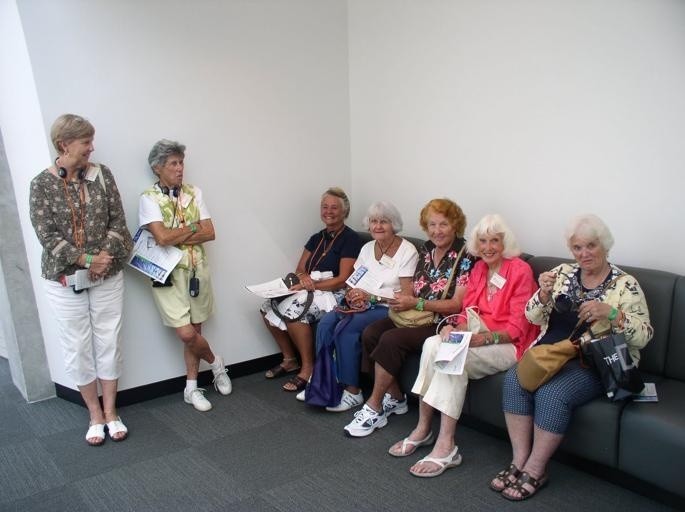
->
<box><xmin>389</xmin><ymin>430</ymin><xmax>435</xmax><ymax>456</ymax></box>
<box><xmin>265</xmin><ymin>364</ymin><xmax>302</xmax><ymax>379</ymax></box>
<box><xmin>502</xmin><ymin>471</ymin><xmax>549</xmax><ymax>501</ymax></box>
<box><xmin>282</xmin><ymin>375</ymin><xmax>308</xmax><ymax>392</ymax></box>
<box><xmin>410</xmin><ymin>446</ymin><xmax>462</xmax><ymax>477</ymax></box>
<box><xmin>486</xmin><ymin>464</ymin><xmax>521</xmax><ymax>492</ymax></box>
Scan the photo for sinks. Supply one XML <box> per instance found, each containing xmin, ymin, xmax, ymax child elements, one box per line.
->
<box><xmin>307</xmin><ymin>231</ymin><xmax>685</xmax><ymax>496</ymax></box>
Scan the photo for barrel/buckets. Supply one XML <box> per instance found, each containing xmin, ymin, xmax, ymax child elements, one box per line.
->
<box><xmin>107</xmin><ymin>417</ymin><xmax>129</xmax><ymax>441</ymax></box>
<box><xmin>86</xmin><ymin>419</ymin><xmax>105</xmax><ymax>446</ymax></box>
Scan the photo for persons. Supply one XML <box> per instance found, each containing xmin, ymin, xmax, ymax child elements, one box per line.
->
<box><xmin>28</xmin><ymin>114</ymin><xmax>129</xmax><ymax>446</ymax></box>
<box><xmin>343</xmin><ymin>197</ymin><xmax>472</xmax><ymax>439</ymax></box>
<box><xmin>294</xmin><ymin>200</ymin><xmax>420</xmax><ymax>413</ymax></box>
<box><xmin>137</xmin><ymin>138</ymin><xmax>233</xmax><ymax>412</ymax></box>
<box><xmin>259</xmin><ymin>186</ymin><xmax>360</xmax><ymax>392</ymax></box>
<box><xmin>489</xmin><ymin>215</ymin><xmax>655</xmax><ymax>501</ymax></box>
<box><xmin>387</xmin><ymin>212</ymin><xmax>542</xmax><ymax>478</ymax></box>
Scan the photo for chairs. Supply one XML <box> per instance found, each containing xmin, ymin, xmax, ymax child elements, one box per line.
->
<box><xmin>344</xmin><ymin>404</ymin><xmax>388</xmax><ymax>437</ymax></box>
<box><xmin>296</xmin><ymin>389</ymin><xmax>308</xmax><ymax>401</ymax></box>
<box><xmin>382</xmin><ymin>393</ymin><xmax>408</xmax><ymax>417</ymax></box>
<box><xmin>183</xmin><ymin>387</ymin><xmax>212</xmax><ymax>412</ymax></box>
<box><xmin>325</xmin><ymin>389</ymin><xmax>364</xmax><ymax>412</ymax></box>
<box><xmin>212</xmin><ymin>354</ymin><xmax>232</xmax><ymax>395</ymax></box>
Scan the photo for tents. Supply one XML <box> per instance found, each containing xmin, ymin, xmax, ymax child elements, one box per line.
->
<box><xmin>547</xmin><ymin>271</ymin><xmax>581</xmax><ymax>317</ymax></box>
<box><xmin>321</xmin><ymin>224</ymin><xmax>345</xmax><ymax>241</ymax></box>
<box><xmin>157</xmin><ymin>180</ymin><xmax>182</xmax><ymax>198</ymax></box>
<box><xmin>53</xmin><ymin>156</ymin><xmax>86</xmax><ymax>180</ymax></box>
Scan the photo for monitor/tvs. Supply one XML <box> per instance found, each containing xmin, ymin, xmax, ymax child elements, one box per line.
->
<box><xmin>75</xmin><ymin>268</ymin><xmax>103</xmax><ymax>291</ymax></box>
<box><xmin>633</xmin><ymin>382</ymin><xmax>658</xmax><ymax>402</ymax></box>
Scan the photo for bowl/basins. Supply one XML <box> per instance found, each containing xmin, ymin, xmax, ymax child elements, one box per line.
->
<box><xmin>377</xmin><ymin>237</ymin><xmax>395</xmax><ymax>264</ymax></box>
<box><xmin>579</xmin><ymin>271</ymin><xmax>605</xmax><ymax>299</ymax></box>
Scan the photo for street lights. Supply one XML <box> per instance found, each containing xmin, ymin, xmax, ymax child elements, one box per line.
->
<box><xmin>271</xmin><ymin>272</ymin><xmax>314</xmax><ymax>322</ymax></box>
<box><xmin>517</xmin><ymin>274</ymin><xmax>628</xmax><ymax>392</ymax></box>
<box><xmin>584</xmin><ymin>322</ymin><xmax>645</xmax><ymax>402</ymax></box>
<box><xmin>94</xmin><ymin>161</ymin><xmax>134</xmax><ymax>268</ymax></box>
<box><xmin>388</xmin><ymin>244</ymin><xmax>466</xmax><ymax>328</ymax></box>
<box><xmin>304</xmin><ymin>311</ymin><xmax>353</xmax><ymax>407</ymax></box>
<box><xmin>437</xmin><ymin>306</ymin><xmax>491</xmax><ymax>337</ymax></box>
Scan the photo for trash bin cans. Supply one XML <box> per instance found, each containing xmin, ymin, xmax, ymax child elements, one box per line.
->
<box><xmin>377</xmin><ymin>296</ymin><xmax>382</xmax><ymax>302</ymax></box>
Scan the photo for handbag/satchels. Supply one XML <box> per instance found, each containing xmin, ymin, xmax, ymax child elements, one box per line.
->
<box><xmin>483</xmin><ymin>332</ymin><xmax>499</xmax><ymax>345</ymax></box>
<box><xmin>370</xmin><ymin>294</ymin><xmax>376</xmax><ymax>304</ymax></box>
<box><xmin>189</xmin><ymin>223</ymin><xmax>197</xmax><ymax>236</ymax></box>
<box><xmin>416</xmin><ymin>297</ymin><xmax>425</xmax><ymax>312</ymax></box>
<box><xmin>608</xmin><ymin>307</ymin><xmax>619</xmax><ymax>321</ymax></box>
<box><xmin>85</xmin><ymin>253</ymin><xmax>92</xmax><ymax>268</ymax></box>
